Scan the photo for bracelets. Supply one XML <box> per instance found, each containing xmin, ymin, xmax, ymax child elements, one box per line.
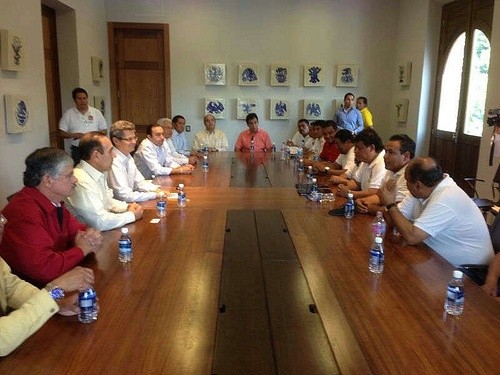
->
<box><xmin>386</xmin><ymin>203</ymin><xmax>396</xmax><ymax>210</ymax></box>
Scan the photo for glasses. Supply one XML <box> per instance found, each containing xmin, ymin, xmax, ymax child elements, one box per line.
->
<box><xmin>114</xmin><ymin>136</ymin><xmax>138</xmax><ymax>141</ymax></box>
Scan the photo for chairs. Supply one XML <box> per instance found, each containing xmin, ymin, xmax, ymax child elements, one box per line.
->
<box><xmin>462</xmin><ymin>177</ymin><xmax>500</xmax><ymax>223</ymax></box>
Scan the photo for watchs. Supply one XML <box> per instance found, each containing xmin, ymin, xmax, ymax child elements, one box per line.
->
<box><xmin>46</xmin><ymin>282</ymin><xmax>64</xmax><ymax>302</ymax></box>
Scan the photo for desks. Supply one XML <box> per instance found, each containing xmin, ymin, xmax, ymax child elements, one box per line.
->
<box><xmin>0</xmin><ymin>150</ymin><xmax>500</xmax><ymax>375</ymax></box>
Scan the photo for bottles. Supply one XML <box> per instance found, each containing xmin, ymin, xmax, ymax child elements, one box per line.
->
<box><xmin>370</xmin><ymin>209</ymin><xmax>388</xmax><ymax>251</ymax></box>
<box><xmin>345</xmin><ymin>193</ymin><xmax>355</xmax><ymax>218</ymax></box>
<box><xmin>369</xmin><ymin>235</ymin><xmax>384</xmax><ymax>273</ymax></box>
<box><xmin>280</xmin><ymin>141</ymin><xmax>286</xmax><ymax>160</ymax></box>
<box><xmin>199</xmin><ymin>141</ymin><xmax>209</xmax><ymax>153</ymax></box>
<box><xmin>202</xmin><ymin>155</ymin><xmax>210</xmax><ymax>173</ymax></box>
<box><xmin>76</xmin><ymin>283</ymin><xmax>98</xmax><ymax>324</ymax></box>
<box><xmin>309</xmin><ymin>177</ymin><xmax>319</xmax><ymax>201</ymax></box>
<box><xmin>306</xmin><ymin>166</ymin><xmax>314</xmax><ymax>184</ymax></box>
<box><xmin>444</xmin><ymin>269</ymin><xmax>466</xmax><ymax>316</ymax></box>
<box><xmin>177</xmin><ymin>183</ymin><xmax>186</xmax><ymax>207</ymax></box>
<box><xmin>249</xmin><ymin>141</ymin><xmax>255</xmax><ymax>152</ymax></box>
<box><xmin>157</xmin><ymin>191</ymin><xmax>167</xmax><ymax>216</ymax></box>
<box><xmin>118</xmin><ymin>228</ymin><xmax>133</xmax><ymax>263</ymax></box>
<box><xmin>299</xmin><ymin>140</ymin><xmax>319</xmax><ymax>160</ymax></box>
<box><xmin>297</xmin><ymin>159</ymin><xmax>305</xmax><ymax>175</ymax></box>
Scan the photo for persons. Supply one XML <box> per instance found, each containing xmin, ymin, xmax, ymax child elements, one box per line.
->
<box><xmin>356</xmin><ymin>134</ymin><xmax>416</xmax><ymax>214</ymax></box>
<box><xmin>0</xmin><ymin>147</ymin><xmax>104</xmax><ymax>283</ymax></box>
<box><xmin>332</xmin><ymin>92</ymin><xmax>363</xmax><ymax>135</ymax></box>
<box><xmin>156</xmin><ymin>115</ymin><xmax>203</xmax><ymax>164</ymax></box>
<box><xmin>235</xmin><ymin>113</ymin><xmax>272</xmax><ymax>152</ymax></box>
<box><xmin>303</xmin><ymin>120</ymin><xmax>340</xmax><ymax>171</ymax></box>
<box><xmin>106</xmin><ymin>120</ymin><xmax>169</xmax><ymax>202</ymax></box>
<box><xmin>288</xmin><ymin>118</ymin><xmax>325</xmax><ymax>158</ymax></box>
<box><xmin>356</xmin><ymin>97</ymin><xmax>373</xmax><ymax>128</ymax></box>
<box><xmin>483</xmin><ymin>249</ymin><xmax>500</xmax><ymax>303</ymax></box>
<box><xmin>315</xmin><ymin>129</ymin><xmax>363</xmax><ymax>184</ymax></box>
<box><xmin>0</xmin><ymin>212</ymin><xmax>99</xmax><ymax>357</ymax></box>
<box><xmin>63</xmin><ymin>132</ymin><xmax>143</xmax><ymax>231</ymax></box>
<box><xmin>135</xmin><ymin>124</ymin><xmax>194</xmax><ymax>179</ymax></box>
<box><xmin>59</xmin><ymin>87</ymin><xmax>108</xmax><ymax>166</ymax></box>
<box><xmin>378</xmin><ymin>157</ymin><xmax>495</xmax><ymax>285</ymax></box>
<box><xmin>192</xmin><ymin>114</ymin><xmax>228</xmax><ymax>152</ymax></box>
<box><xmin>336</xmin><ymin>128</ymin><xmax>394</xmax><ymax>199</ymax></box>
